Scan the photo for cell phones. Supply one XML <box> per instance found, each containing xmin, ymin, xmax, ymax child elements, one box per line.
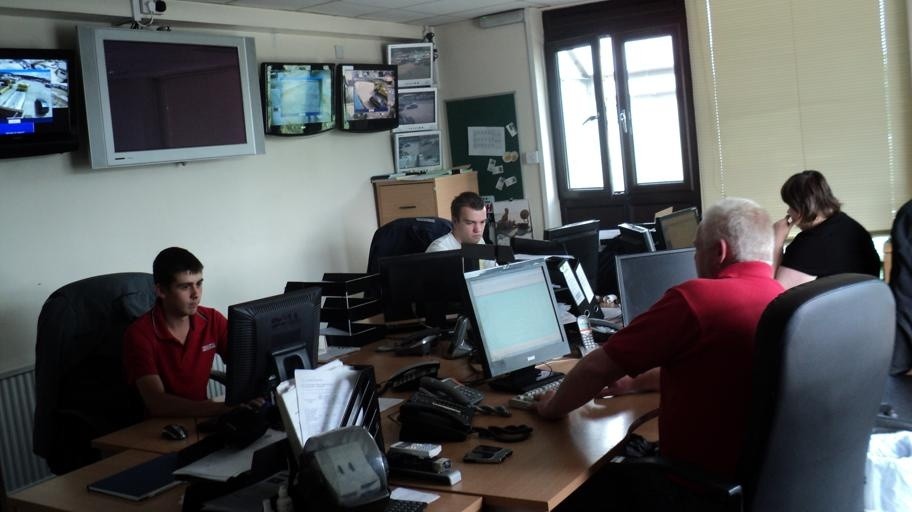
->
<box><xmin>463</xmin><ymin>450</ymin><xmax>506</xmax><ymax>464</ymax></box>
<box><xmin>473</xmin><ymin>444</ymin><xmax>513</xmax><ymax>455</ymax></box>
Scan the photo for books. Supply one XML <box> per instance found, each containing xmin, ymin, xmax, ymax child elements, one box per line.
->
<box><xmin>86</xmin><ymin>453</ymin><xmax>181</xmax><ymax>503</ymax></box>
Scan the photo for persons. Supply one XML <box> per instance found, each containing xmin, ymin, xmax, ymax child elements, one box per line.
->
<box><xmin>424</xmin><ymin>191</ymin><xmax>487</xmax><ymax>255</ymax></box>
<box><xmin>115</xmin><ymin>247</ymin><xmax>265</xmax><ymax>420</ymax></box>
<box><xmin>773</xmin><ymin>170</ymin><xmax>880</xmax><ymax>291</ymax></box>
<box><xmin>526</xmin><ymin>198</ymin><xmax>785</xmax><ymax>511</ymax></box>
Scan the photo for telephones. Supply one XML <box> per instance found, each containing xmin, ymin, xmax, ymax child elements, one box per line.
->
<box><xmin>396</xmin><ymin>325</ymin><xmax>442</xmax><ymax>356</ymax></box>
<box><xmin>376</xmin><ymin>359</ymin><xmax>440</xmax><ymax>396</ymax></box>
<box><xmin>443</xmin><ymin>316</ymin><xmax>473</xmax><ymax>360</ymax></box>
<box><xmin>574</xmin><ymin>311</ymin><xmax>599</xmax><ymax>357</ymax></box>
<box><xmin>398</xmin><ymin>391</ymin><xmax>534</xmax><ymax>445</ymax></box>
<box><xmin>589</xmin><ymin>318</ymin><xmax>624</xmax><ymax>344</ymax></box>
<box><xmin>419</xmin><ymin>376</ymin><xmax>512</xmax><ymax>417</ymax></box>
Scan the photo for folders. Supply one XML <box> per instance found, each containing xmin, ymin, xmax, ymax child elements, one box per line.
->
<box><xmin>568</xmin><ymin>257</ymin><xmax>604</xmax><ymax>319</ymax></box>
<box><xmin>546</xmin><ymin>257</ymin><xmax>598</xmax><ymax>319</ymax></box>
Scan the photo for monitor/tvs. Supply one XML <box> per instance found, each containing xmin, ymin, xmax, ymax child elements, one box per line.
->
<box><xmin>337</xmin><ymin>64</ymin><xmax>400</xmax><ymax>133</ymax></box>
<box><xmin>619</xmin><ymin>222</ymin><xmax>656</xmax><ymax>251</ymax></box>
<box><xmin>392</xmin><ymin>87</ymin><xmax>439</xmax><ymax>132</ymax></box>
<box><xmin>655</xmin><ymin>206</ymin><xmax>700</xmax><ymax>250</ymax></box>
<box><xmin>261</xmin><ymin>62</ymin><xmax>336</xmax><ymax>137</ymax></box>
<box><xmin>387</xmin><ymin>42</ymin><xmax>433</xmax><ymax>87</ymax></box>
<box><xmin>543</xmin><ymin>219</ymin><xmax>600</xmax><ymax>293</ymax></box>
<box><xmin>394</xmin><ymin>131</ymin><xmax>442</xmax><ymax>174</ymax></box>
<box><xmin>223</xmin><ymin>287</ymin><xmax>321</xmax><ymax>428</ymax></box>
<box><xmin>76</xmin><ymin>25</ymin><xmax>266</xmax><ymax>170</ymax></box>
<box><xmin>378</xmin><ymin>249</ymin><xmax>463</xmax><ymax>339</ymax></box>
<box><xmin>613</xmin><ymin>248</ymin><xmax>696</xmax><ymax>328</ymax></box>
<box><xmin>462</xmin><ymin>258</ymin><xmax>571</xmax><ymax>394</ymax></box>
<box><xmin>0</xmin><ymin>47</ymin><xmax>79</xmax><ymax>161</ymax></box>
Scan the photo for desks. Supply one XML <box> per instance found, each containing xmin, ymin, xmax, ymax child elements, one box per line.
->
<box><xmin>9</xmin><ymin>449</ymin><xmax>482</xmax><ymax>511</ymax></box>
<box><xmin>89</xmin><ymin>349</ymin><xmax>661</xmax><ymax>512</ymax></box>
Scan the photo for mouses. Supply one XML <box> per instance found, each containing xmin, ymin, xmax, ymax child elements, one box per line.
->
<box><xmin>161</xmin><ymin>423</ymin><xmax>187</xmax><ymax>440</ymax></box>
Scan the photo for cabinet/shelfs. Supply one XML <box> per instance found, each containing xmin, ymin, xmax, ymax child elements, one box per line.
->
<box><xmin>374</xmin><ymin>171</ymin><xmax>478</xmax><ymax>228</ymax></box>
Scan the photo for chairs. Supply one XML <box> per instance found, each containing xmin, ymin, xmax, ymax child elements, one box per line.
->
<box><xmin>710</xmin><ymin>274</ymin><xmax>897</xmax><ymax>511</ymax></box>
<box><xmin>33</xmin><ymin>273</ymin><xmax>158</xmax><ymax>474</ymax></box>
<box><xmin>364</xmin><ymin>216</ymin><xmax>452</xmax><ymax>321</ymax></box>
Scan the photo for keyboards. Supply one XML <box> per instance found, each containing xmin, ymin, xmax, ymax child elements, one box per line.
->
<box><xmin>361</xmin><ymin>499</ymin><xmax>429</xmax><ymax>512</ymax></box>
<box><xmin>508</xmin><ymin>376</ymin><xmax>564</xmax><ymax>408</ymax></box>
<box><xmin>198</xmin><ymin>401</ymin><xmax>270</xmax><ymax>434</ymax></box>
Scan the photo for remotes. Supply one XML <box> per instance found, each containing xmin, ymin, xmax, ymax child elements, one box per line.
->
<box><xmin>390</xmin><ymin>441</ymin><xmax>442</xmax><ymax>458</ymax></box>
